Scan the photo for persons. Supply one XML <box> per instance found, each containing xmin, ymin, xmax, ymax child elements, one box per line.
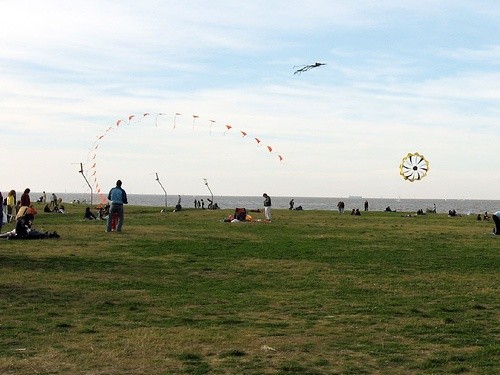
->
<box><xmin>477</xmin><ymin>214</ymin><xmax>482</xmax><ymax>221</ymax></box>
<box><xmin>337</xmin><ymin>200</ymin><xmax>342</xmax><ymax>212</ymax></box>
<box><xmin>263</xmin><ymin>193</ymin><xmax>272</xmax><ymax>222</ymax></box>
<box><xmin>7</xmin><ymin>189</ymin><xmax>16</xmax><ymax>223</ymax></box>
<box><xmin>16</xmin><ymin>213</ymin><xmax>60</xmax><ymax>239</ymax></box>
<box><xmin>484</xmin><ymin>211</ymin><xmax>491</xmax><ymax>222</ymax></box>
<box><xmin>433</xmin><ymin>203</ymin><xmax>437</xmax><ymax>213</ymax></box>
<box><xmin>350</xmin><ymin>209</ymin><xmax>356</xmax><ymax>215</ymax></box>
<box><xmin>355</xmin><ymin>209</ymin><xmax>361</xmax><ymax>216</ymax></box>
<box><xmin>83</xmin><ymin>207</ymin><xmax>97</xmax><ymax>220</ymax></box>
<box><xmin>58</xmin><ymin>205</ymin><xmax>64</xmax><ymax>213</ymax></box>
<box><xmin>49</xmin><ymin>193</ymin><xmax>55</xmax><ymax>206</ymax></box>
<box><xmin>52</xmin><ymin>205</ymin><xmax>59</xmax><ymax>212</ymax></box>
<box><xmin>176</xmin><ymin>195</ymin><xmax>181</xmax><ymax>205</ymax></box>
<box><xmin>105</xmin><ymin>179</ymin><xmax>128</xmax><ymax>233</ymax></box>
<box><xmin>27</xmin><ymin>205</ymin><xmax>38</xmax><ymax>214</ymax></box>
<box><xmin>20</xmin><ymin>187</ymin><xmax>31</xmax><ymax>207</ymax></box>
<box><xmin>43</xmin><ymin>204</ymin><xmax>52</xmax><ymax>212</ymax></box>
<box><xmin>341</xmin><ymin>202</ymin><xmax>345</xmax><ymax>213</ymax></box>
<box><xmin>492</xmin><ymin>210</ymin><xmax>500</xmax><ymax>235</ymax></box>
<box><xmin>365</xmin><ymin>200</ymin><xmax>368</xmax><ymax>211</ymax></box>
<box><xmin>288</xmin><ymin>198</ymin><xmax>295</xmax><ymax>210</ymax></box>
<box><xmin>42</xmin><ymin>191</ymin><xmax>47</xmax><ymax>202</ymax></box>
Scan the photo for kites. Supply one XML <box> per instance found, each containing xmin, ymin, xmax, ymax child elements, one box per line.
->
<box><xmin>267</xmin><ymin>145</ymin><xmax>273</xmax><ymax>152</ymax></box>
<box><xmin>144</xmin><ymin>112</ymin><xmax>150</xmax><ymax>116</ymax></box>
<box><xmin>240</xmin><ymin>131</ymin><xmax>248</xmax><ymax>137</ymax></box>
<box><xmin>128</xmin><ymin>115</ymin><xmax>135</xmax><ymax>122</ymax></box>
<box><xmin>116</xmin><ymin>119</ymin><xmax>123</xmax><ymax>126</ymax></box>
<box><xmin>399</xmin><ymin>152</ymin><xmax>430</xmax><ymax>183</ymax></box>
<box><xmin>277</xmin><ymin>154</ymin><xmax>283</xmax><ymax>161</ymax></box>
<box><xmin>225</xmin><ymin>124</ymin><xmax>232</xmax><ymax>130</ymax></box>
<box><xmin>155</xmin><ymin>112</ymin><xmax>165</xmax><ymax>126</ymax></box>
<box><xmin>292</xmin><ymin>62</ymin><xmax>328</xmax><ymax>75</ymax></box>
<box><xmin>254</xmin><ymin>137</ymin><xmax>261</xmax><ymax>143</ymax></box>
<box><xmin>193</xmin><ymin>114</ymin><xmax>200</xmax><ymax>120</ymax></box>
<box><xmin>106</xmin><ymin>126</ymin><xmax>112</xmax><ymax>132</ymax></box>
<box><xmin>211</xmin><ymin>119</ymin><xmax>216</xmax><ymax>123</ymax></box>
<box><xmin>90</xmin><ymin>133</ymin><xmax>105</xmax><ymax>202</ymax></box>
<box><xmin>173</xmin><ymin>113</ymin><xmax>182</xmax><ymax>129</ymax></box>
